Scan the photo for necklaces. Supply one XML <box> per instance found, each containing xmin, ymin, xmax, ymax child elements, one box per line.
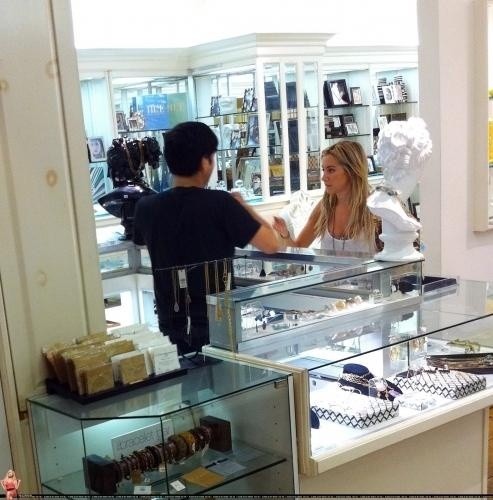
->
<box><xmin>376</xmin><ymin>186</ymin><xmax>413</xmax><ymax>217</ymax></box>
<box><xmin>168</xmin><ymin>256</ymin><xmax>237</xmax><ymax>362</ymax></box>
<box><xmin>341</xmin><ymin>372</ymin><xmax>388</xmax><ymax>392</ymax></box>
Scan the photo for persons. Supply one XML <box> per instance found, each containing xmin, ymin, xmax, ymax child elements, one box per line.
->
<box><xmin>1</xmin><ymin>470</ymin><xmax>21</xmax><ymax>500</ymax></box>
<box><xmin>132</xmin><ymin>121</ymin><xmax>287</xmax><ymax>405</ymax></box>
<box><xmin>271</xmin><ymin>139</ymin><xmax>384</xmax><ymax>254</ymax></box>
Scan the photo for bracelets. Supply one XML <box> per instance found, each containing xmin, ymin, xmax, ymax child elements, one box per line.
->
<box><xmin>110</xmin><ymin>426</ymin><xmax>212</xmax><ymax>483</ymax></box>
<box><xmin>280</xmin><ymin>231</ymin><xmax>291</xmax><ymax>240</ymax></box>
